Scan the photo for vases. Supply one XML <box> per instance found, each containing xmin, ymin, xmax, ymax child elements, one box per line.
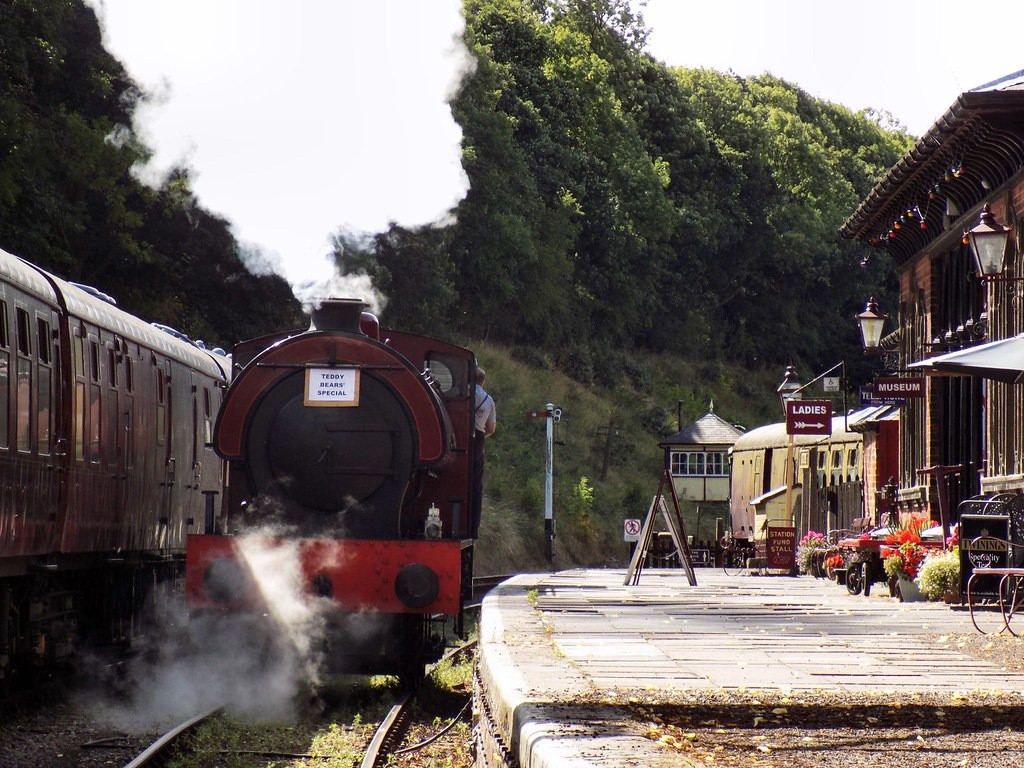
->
<box><xmin>826</xmin><ymin>567</ymin><xmax>836</xmax><ymax>579</ymax></box>
<box><xmin>898</xmin><ymin>579</ymin><xmax>926</xmax><ymax>602</ymax></box>
<box><xmin>944</xmin><ymin>594</ymin><xmax>960</xmax><ymax>603</ymax></box>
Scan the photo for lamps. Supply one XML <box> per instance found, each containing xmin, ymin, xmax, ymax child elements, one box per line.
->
<box><xmin>966</xmin><ymin>204</ymin><xmax>1023</xmax><ymax>286</ymax></box>
<box><xmin>854</xmin><ymin>297</ymin><xmax>900</xmax><ymax>354</ymax></box>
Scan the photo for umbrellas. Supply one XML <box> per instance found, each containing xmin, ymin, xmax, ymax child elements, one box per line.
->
<box><xmin>906</xmin><ymin>332</ymin><xmax>1024</xmax><ymax>384</ymax></box>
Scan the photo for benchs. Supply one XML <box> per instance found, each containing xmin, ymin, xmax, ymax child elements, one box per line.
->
<box><xmin>967</xmin><ymin>536</ymin><xmax>1024</xmax><ymax>637</ymax></box>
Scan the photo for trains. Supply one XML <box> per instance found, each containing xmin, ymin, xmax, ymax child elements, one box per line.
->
<box><xmin>0</xmin><ymin>244</ymin><xmax>496</xmax><ymax>695</ymax></box>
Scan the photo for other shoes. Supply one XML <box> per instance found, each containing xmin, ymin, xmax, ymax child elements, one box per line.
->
<box><xmin>471</xmin><ymin>528</ymin><xmax>478</xmax><ymax>539</ymax></box>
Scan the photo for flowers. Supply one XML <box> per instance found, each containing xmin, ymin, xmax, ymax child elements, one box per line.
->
<box><xmin>879</xmin><ymin>514</ymin><xmax>961</xmax><ymax>600</ymax></box>
<box><xmin>795</xmin><ymin>531</ymin><xmax>844</xmax><ymax>572</ymax></box>
